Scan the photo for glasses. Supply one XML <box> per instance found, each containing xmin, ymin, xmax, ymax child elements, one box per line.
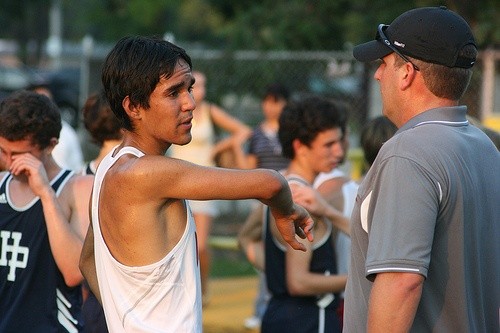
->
<box><xmin>375</xmin><ymin>23</ymin><xmax>420</xmax><ymax>71</ymax></box>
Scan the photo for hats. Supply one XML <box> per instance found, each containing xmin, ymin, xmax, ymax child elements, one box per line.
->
<box><xmin>353</xmin><ymin>6</ymin><xmax>478</xmax><ymax>69</ymax></box>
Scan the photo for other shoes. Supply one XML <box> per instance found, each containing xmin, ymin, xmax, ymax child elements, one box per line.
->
<box><xmin>243</xmin><ymin>316</ymin><xmax>261</xmax><ymax>328</ymax></box>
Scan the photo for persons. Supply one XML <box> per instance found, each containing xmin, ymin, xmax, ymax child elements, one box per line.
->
<box><xmin>260</xmin><ymin>96</ymin><xmax>347</xmax><ymax>333</ymax></box>
<box><xmin>171</xmin><ymin>72</ymin><xmax>254</xmax><ymax>308</ymax></box>
<box><xmin>31</xmin><ymin>83</ymin><xmax>86</xmax><ymax>174</ymax></box>
<box><xmin>91</xmin><ymin>33</ymin><xmax>314</xmax><ymax>333</ymax></box>
<box><xmin>344</xmin><ymin>6</ymin><xmax>500</xmax><ymax>333</ymax></box>
<box><xmin>244</xmin><ymin>82</ymin><xmax>286</xmax><ymax>330</ymax></box>
<box><xmin>321</xmin><ymin>115</ymin><xmax>400</xmax><ymax>333</ymax></box>
<box><xmin>1</xmin><ymin>92</ymin><xmax>94</xmax><ymax>333</ymax></box>
<box><xmin>74</xmin><ymin>94</ymin><xmax>131</xmax><ymax>176</ymax></box>
